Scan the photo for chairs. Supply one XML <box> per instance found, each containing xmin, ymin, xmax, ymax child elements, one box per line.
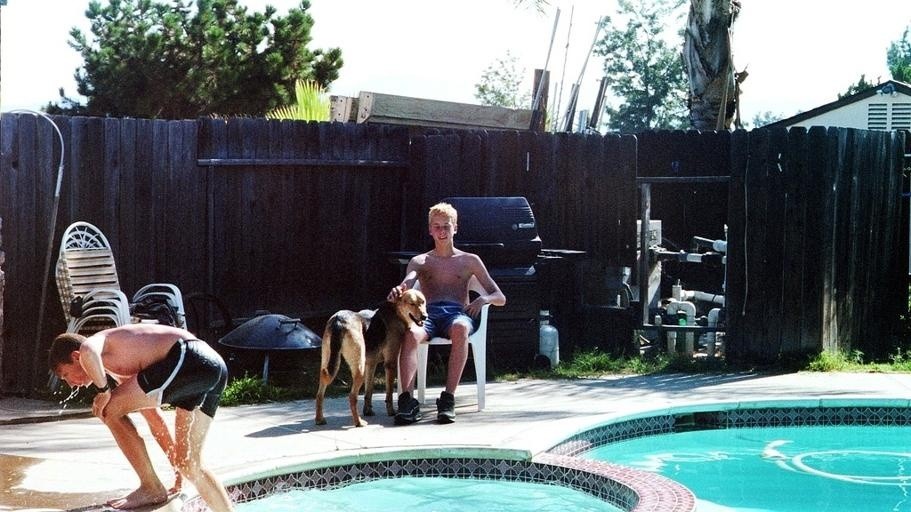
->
<box><xmin>396</xmin><ymin>274</ymin><xmax>491</xmax><ymax>413</ymax></box>
<box><xmin>46</xmin><ymin>221</ymin><xmax>186</xmax><ymax>394</ymax></box>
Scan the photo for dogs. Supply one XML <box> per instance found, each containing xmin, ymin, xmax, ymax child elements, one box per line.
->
<box><xmin>315</xmin><ymin>289</ymin><xmax>428</xmax><ymax>427</ymax></box>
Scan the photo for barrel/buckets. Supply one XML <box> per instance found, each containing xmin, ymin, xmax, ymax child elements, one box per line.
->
<box><xmin>539</xmin><ymin>310</ymin><xmax>560</xmax><ymax>369</ymax></box>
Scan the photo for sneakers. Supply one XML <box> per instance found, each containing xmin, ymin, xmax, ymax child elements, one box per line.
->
<box><xmin>394</xmin><ymin>391</ymin><xmax>422</xmax><ymax>424</ymax></box>
<box><xmin>435</xmin><ymin>390</ymin><xmax>456</xmax><ymax>423</ymax></box>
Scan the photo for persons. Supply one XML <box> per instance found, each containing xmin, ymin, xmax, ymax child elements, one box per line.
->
<box><xmin>387</xmin><ymin>202</ymin><xmax>506</xmax><ymax>427</ymax></box>
<box><xmin>48</xmin><ymin>323</ymin><xmax>232</xmax><ymax>512</ymax></box>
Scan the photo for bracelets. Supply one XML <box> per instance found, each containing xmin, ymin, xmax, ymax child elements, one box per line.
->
<box><xmin>95</xmin><ymin>383</ymin><xmax>110</xmax><ymax>394</ymax></box>
